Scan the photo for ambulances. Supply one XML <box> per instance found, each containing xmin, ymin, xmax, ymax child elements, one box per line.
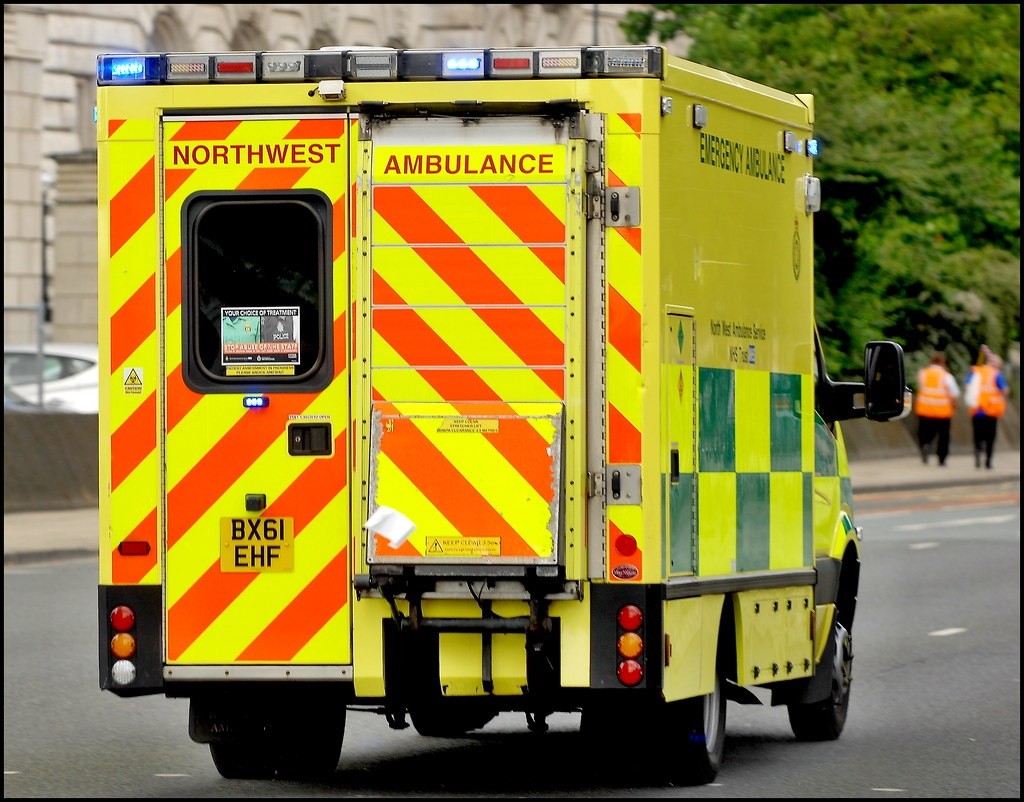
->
<box><xmin>91</xmin><ymin>44</ymin><xmax>905</xmax><ymax>790</ymax></box>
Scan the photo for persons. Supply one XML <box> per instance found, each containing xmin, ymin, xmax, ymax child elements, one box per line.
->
<box><xmin>913</xmin><ymin>351</ymin><xmax>959</xmax><ymax>466</ymax></box>
<box><xmin>965</xmin><ymin>354</ymin><xmax>1009</xmax><ymax>469</ymax></box>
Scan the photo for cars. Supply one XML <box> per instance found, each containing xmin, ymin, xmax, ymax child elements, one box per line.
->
<box><xmin>4</xmin><ymin>345</ymin><xmax>98</xmax><ymax>411</ymax></box>
